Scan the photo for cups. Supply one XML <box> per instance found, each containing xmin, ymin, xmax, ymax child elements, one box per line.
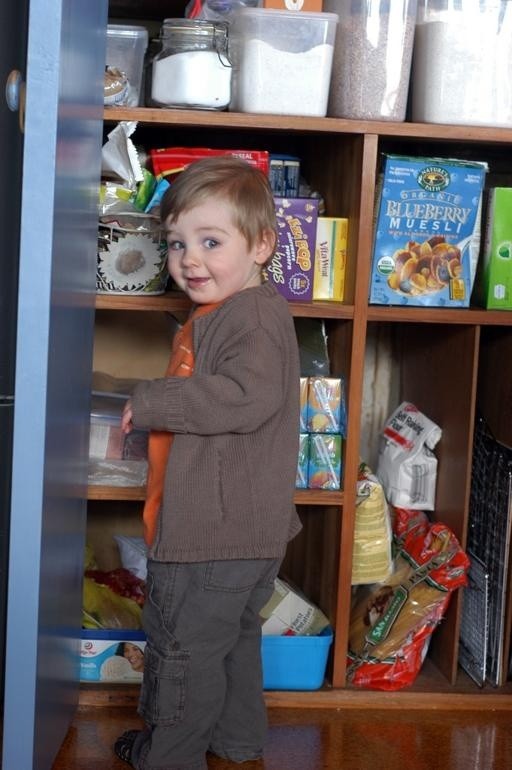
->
<box><xmin>327</xmin><ymin>0</ymin><xmax>505</xmax><ymax>130</ymax></box>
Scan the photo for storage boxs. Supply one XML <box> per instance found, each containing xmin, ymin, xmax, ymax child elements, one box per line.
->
<box><xmin>250</xmin><ymin>631</ymin><xmax>334</xmax><ymax>698</ymax></box>
<box><xmin>80</xmin><ymin>620</ymin><xmax>150</xmax><ymax>684</ymax></box>
<box><xmin>87</xmin><ymin>370</ymin><xmax>153</xmax><ymax>462</ymax></box>
<box><xmin>370</xmin><ymin>149</ymin><xmax>490</xmax><ymax>307</ymax></box>
<box><xmin>256</xmin><ymin>192</ymin><xmax>323</xmax><ymax>304</ymax></box>
<box><xmin>230</xmin><ymin>10</ymin><xmax>338</xmax><ymax>117</ymax></box>
<box><xmin>412</xmin><ymin>1</ymin><xmax>509</xmax><ymax>131</ymax></box>
<box><xmin>323</xmin><ymin>1</ymin><xmax>416</xmax><ymax>119</ymax></box>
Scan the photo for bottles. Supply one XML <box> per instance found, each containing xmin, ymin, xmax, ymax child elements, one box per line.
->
<box><xmin>151</xmin><ymin>19</ymin><xmax>232</xmax><ymax>110</ymax></box>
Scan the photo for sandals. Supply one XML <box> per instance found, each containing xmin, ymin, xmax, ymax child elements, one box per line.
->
<box><xmin>113</xmin><ymin>727</ymin><xmax>146</xmax><ymax>764</ymax></box>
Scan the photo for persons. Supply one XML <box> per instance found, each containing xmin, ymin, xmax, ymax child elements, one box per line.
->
<box><xmin>119</xmin><ymin>151</ymin><xmax>308</xmax><ymax>769</ymax></box>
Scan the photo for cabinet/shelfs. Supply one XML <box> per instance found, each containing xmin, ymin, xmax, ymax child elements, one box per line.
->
<box><xmin>54</xmin><ymin>102</ymin><xmax>512</xmax><ymax>702</ymax></box>
